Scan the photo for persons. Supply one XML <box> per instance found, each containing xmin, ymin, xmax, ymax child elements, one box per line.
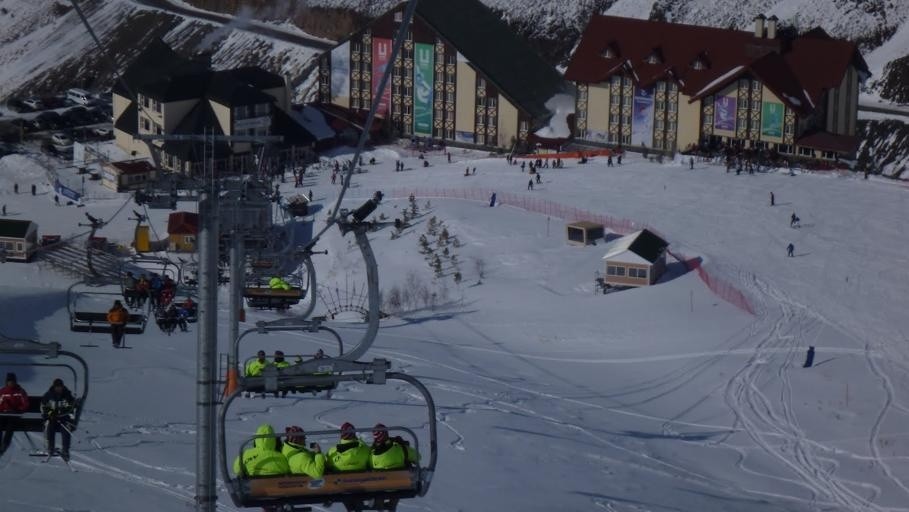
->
<box><xmin>1</xmin><ymin>370</ymin><xmax>29</xmax><ymax>459</ymax></box>
<box><xmin>121</xmin><ymin>269</ymin><xmax>197</xmax><ymax>336</ymax></box>
<box><xmin>325</xmin><ymin>422</ymin><xmax>373</xmax><ymax>512</ymax></box>
<box><xmin>39</xmin><ymin>377</ymin><xmax>81</xmax><ymax>466</ymax></box>
<box><xmin>106</xmin><ymin>298</ymin><xmax>128</xmax><ymax>348</ymax></box>
<box><xmin>279</xmin><ymin>426</ymin><xmax>333</xmax><ymax>507</ymax></box>
<box><xmin>368</xmin><ymin>424</ymin><xmax>422</xmax><ymax>511</ymax></box>
<box><xmin>246</xmin><ymin>347</ymin><xmax>271</xmax><ymax>400</ymax></box>
<box><xmin>269</xmin><ymin>276</ymin><xmax>291</xmax><ymax>291</ymax></box>
<box><xmin>233</xmin><ymin>423</ymin><xmax>290</xmax><ymax>512</ymax></box>
<box><xmin>291</xmin><ymin>355</ymin><xmax>311</xmax><ymax>396</ymax></box>
<box><xmin>270</xmin><ymin>350</ymin><xmax>291</xmax><ymax>398</ymax></box>
<box><xmin>311</xmin><ymin>348</ymin><xmax>336</xmax><ymax>397</ymax></box>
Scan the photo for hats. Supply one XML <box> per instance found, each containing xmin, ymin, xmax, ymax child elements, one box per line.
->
<box><xmin>6</xmin><ymin>373</ymin><xmax>16</xmax><ymax>381</ymax></box>
<box><xmin>341</xmin><ymin>422</ymin><xmax>356</xmax><ymax>440</ymax></box>
<box><xmin>275</xmin><ymin>350</ymin><xmax>323</xmax><ymax>362</ymax></box>
<box><xmin>373</xmin><ymin>424</ymin><xmax>389</xmax><ymax>441</ymax></box>
<box><xmin>286</xmin><ymin>426</ymin><xmax>304</xmax><ymax>442</ymax></box>
